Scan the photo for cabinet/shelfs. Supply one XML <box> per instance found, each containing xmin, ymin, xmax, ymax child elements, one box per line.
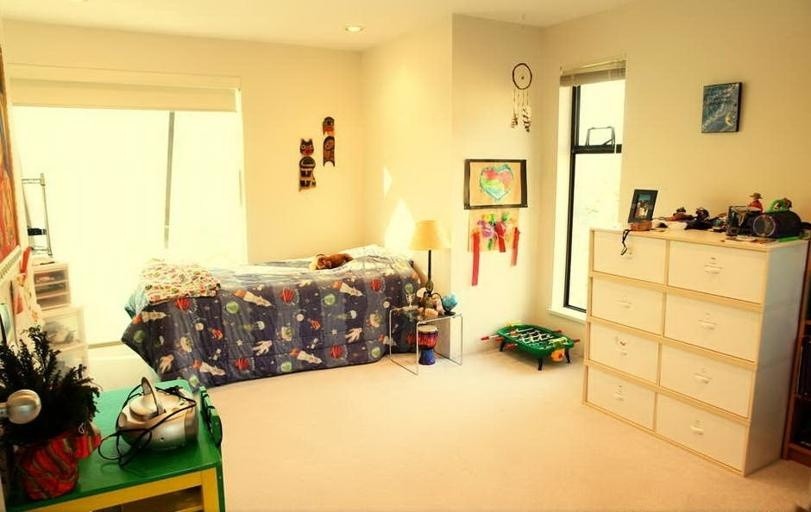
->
<box><xmin>390</xmin><ymin>307</ymin><xmax>465</xmax><ymax>376</ymax></box>
<box><xmin>581</xmin><ymin>217</ymin><xmax>810</xmax><ymax>477</ymax></box>
<box><xmin>30</xmin><ymin>261</ymin><xmax>87</xmax><ymax>374</ymax></box>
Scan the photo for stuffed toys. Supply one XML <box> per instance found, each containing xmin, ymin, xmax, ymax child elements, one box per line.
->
<box><xmin>309</xmin><ymin>252</ymin><xmax>354</xmax><ymax>270</ymax></box>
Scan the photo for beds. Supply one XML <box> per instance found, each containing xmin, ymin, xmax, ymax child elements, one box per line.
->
<box><xmin>133</xmin><ymin>243</ymin><xmax>442</xmax><ymax>388</ymax></box>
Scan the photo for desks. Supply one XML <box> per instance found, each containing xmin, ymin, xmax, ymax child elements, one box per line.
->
<box><xmin>0</xmin><ymin>378</ymin><xmax>220</xmax><ymax>512</ymax></box>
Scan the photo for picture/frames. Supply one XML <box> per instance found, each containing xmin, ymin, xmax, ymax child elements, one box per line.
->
<box><xmin>629</xmin><ymin>188</ymin><xmax>659</xmax><ymax>221</ymax></box>
<box><xmin>464</xmin><ymin>159</ymin><xmax>529</xmax><ymax>208</ymax></box>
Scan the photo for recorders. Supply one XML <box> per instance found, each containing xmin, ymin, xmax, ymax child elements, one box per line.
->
<box><xmin>116</xmin><ymin>377</ymin><xmax>199</xmax><ymax>452</ymax></box>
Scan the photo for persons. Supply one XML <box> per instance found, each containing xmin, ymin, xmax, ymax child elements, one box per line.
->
<box><xmin>749</xmin><ymin>192</ymin><xmax>763</xmax><ymax>213</ymax></box>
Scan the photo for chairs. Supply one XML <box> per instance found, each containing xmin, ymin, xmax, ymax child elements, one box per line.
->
<box><xmin>200</xmin><ymin>387</ymin><xmax>224</xmax><ymax>509</ymax></box>
